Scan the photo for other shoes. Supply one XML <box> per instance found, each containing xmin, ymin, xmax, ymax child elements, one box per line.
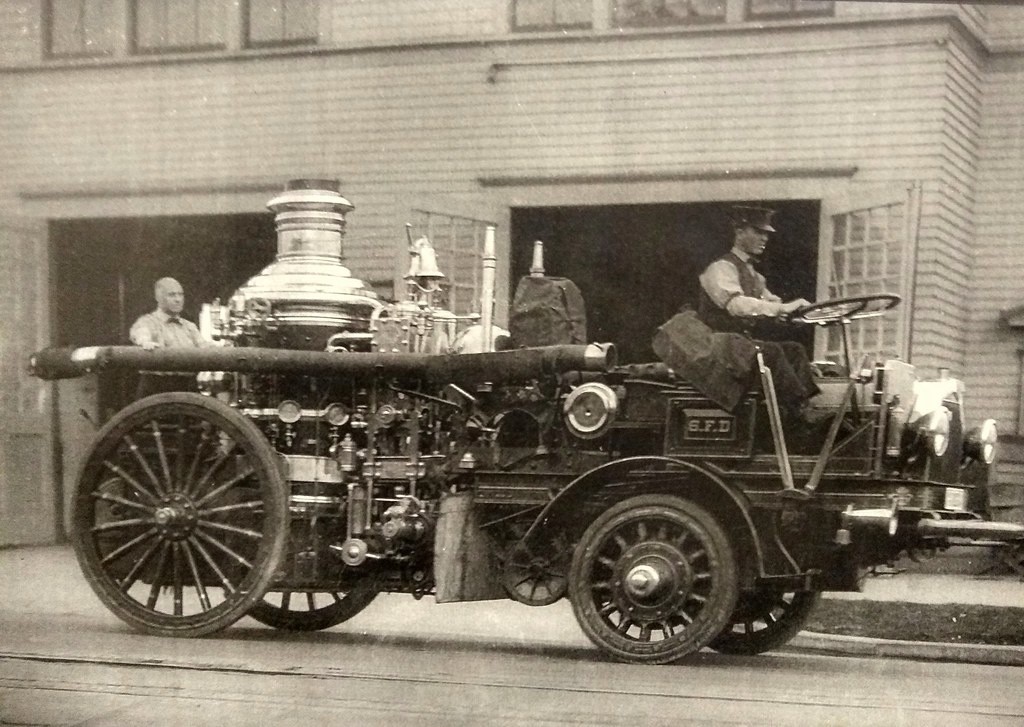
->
<box><xmin>789</xmin><ymin>408</ymin><xmax>835</xmax><ymax>431</ymax></box>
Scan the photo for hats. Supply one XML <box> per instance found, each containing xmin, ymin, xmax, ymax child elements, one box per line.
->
<box><xmin>731</xmin><ymin>204</ymin><xmax>777</xmax><ymax>232</ymax></box>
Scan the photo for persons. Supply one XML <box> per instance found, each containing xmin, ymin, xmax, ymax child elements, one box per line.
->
<box><xmin>698</xmin><ymin>205</ymin><xmax>853</xmax><ymax>438</ymax></box>
<box><xmin>128</xmin><ymin>276</ymin><xmax>221</xmax><ymax>428</ymax></box>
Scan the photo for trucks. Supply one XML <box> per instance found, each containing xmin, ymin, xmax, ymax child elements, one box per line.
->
<box><xmin>26</xmin><ymin>179</ymin><xmax>1024</xmax><ymax>665</ymax></box>
